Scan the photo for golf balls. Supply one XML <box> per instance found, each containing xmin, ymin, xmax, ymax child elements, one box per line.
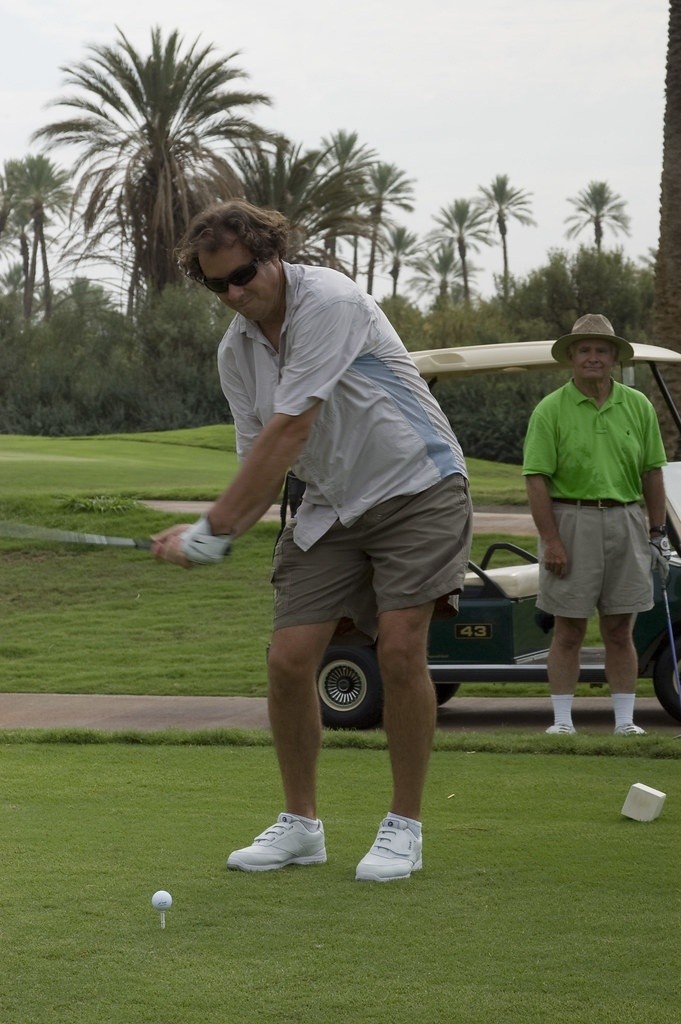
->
<box><xmin>152</xmin><ymin>890</ymin><xmax>173</xmax><ymax>911</ymax></box>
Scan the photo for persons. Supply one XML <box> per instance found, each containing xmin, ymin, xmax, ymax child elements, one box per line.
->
<box><xmin>148</xmin><ymin>202</ymin><xmax>474</xmax><ymax>882</ymax></box>
<box><xmin>522</xmin><ymin>313</ymin><xmax>671</xmax><ymax>738</ymax></box>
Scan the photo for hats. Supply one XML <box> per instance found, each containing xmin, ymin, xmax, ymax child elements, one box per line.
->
<box><xmin>551</xmin><ymin>314</ymin><xmax>634</xmax><ymax>366</ymax></box>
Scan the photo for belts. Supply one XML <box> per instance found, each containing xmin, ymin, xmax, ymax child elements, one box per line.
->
<box><xmin>551</xmin><ymin>498</ymin><xmax>638</xmax><ymax>509</ymax></box>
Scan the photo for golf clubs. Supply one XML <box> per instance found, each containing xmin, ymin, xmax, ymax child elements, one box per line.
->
<box><xmin>0</xmin><ymin>520</ymin><xmax>234</xmax><ymax>557</ymax></box>
<box><xmin>658</xmin><ymin>560</ymin><xmax>681</xmax><ymax>706</ymax></box>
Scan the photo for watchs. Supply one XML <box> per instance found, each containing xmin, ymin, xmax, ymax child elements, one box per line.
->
<box><xmin>650</xmin><ymin>524</ymin><xmax>666</xmax><ymax>536</ymax></box>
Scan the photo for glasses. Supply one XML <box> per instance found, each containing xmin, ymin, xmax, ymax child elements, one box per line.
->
<box><xmin>201</xmin><ymin>254</ymin><xmax>260</xmax><ymax>293</ymax></box>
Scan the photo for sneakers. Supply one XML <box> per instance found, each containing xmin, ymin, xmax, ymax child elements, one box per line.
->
<box><xmin>545</xmin><ymin>725</ymin><xmax>576</xmax><ymax>735</ymax></box>
<box><xmin>356</xmin><ymin>817</ymin><xmax>423</xmax><ymax>882</ymax></box>
<box><xmin>615</xmin><ymin>722</ymin><xmax>647</xmax><ymax>735</ymax></box>
<box><xmin>227</xmin><ymin>812</ymin><xmax>327</xmax><ymax>872</ymax></box>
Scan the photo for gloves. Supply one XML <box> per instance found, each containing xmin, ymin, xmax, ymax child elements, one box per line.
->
<box><xmin>648</xmin><ymin>534</ymin><xmax>671</xmax><ymax>580</ymax></box>
<box><xmin>166</xmin><ymin>513</ymin><xmax>232</xmax><ymax>565</ymax></box>
